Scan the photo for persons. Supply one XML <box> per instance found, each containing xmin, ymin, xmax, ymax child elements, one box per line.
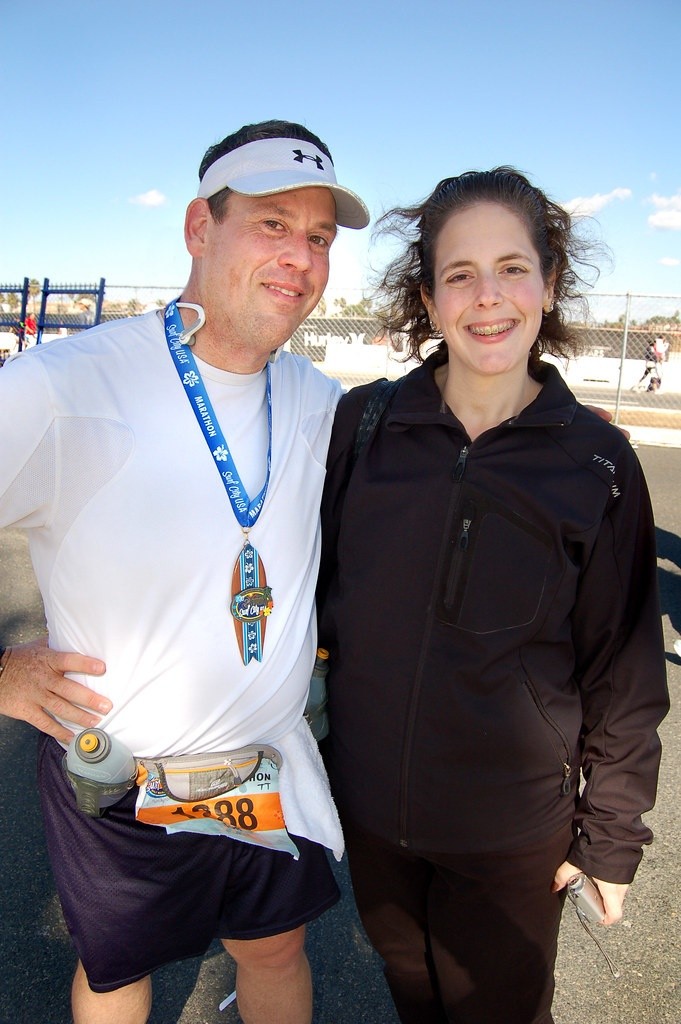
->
<box><xmin>9</xmin><ymin>311</ymin><xmax>39</xmax><ymax>356</ymax></box>
<box><xmin>628</xmin><ymin>333</ymin><xmax>670</xmax><ymax>394</ymax></box>
<box><xmin>1</xmin><ymin>120</ymin><xmax>371</xmax><ymax>1024</ymax></box>
<box><xmin>319</xmin><ymin>163</ymin><xmax>673</xmax><ymax>1024</ymax></box>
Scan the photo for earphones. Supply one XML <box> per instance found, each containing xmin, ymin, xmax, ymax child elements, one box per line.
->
<box><xmin>268</xmin><ymin>350</ymin><xmax>277</xmax><ymax>363</ymax></box>
<box><xmin>179</xmin><ymin>329</ymin><xmax>196</xmax><ymax>346</ymax></box>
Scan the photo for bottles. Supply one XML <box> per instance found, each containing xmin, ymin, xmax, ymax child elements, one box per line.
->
<box><xmin>68</xmin><ymin>730</ymin><xmax>135</xmax><ymax>812</ymax></box>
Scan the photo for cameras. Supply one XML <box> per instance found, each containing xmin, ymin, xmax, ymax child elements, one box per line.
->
<box><xmin>567</xmin><ymin>872</ymin><xmax>605</xmax><ymax>923</ymax></box>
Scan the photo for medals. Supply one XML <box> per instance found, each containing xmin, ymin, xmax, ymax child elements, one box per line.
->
<box><xmin>230</xmin><ymin>543</ymin><xmax>273</xmax><ymax>667</ymax></box>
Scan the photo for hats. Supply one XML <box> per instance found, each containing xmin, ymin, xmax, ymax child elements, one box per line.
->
<box><xmin>196</xmin><ymin>137</ymin><xmax>370</xmax><ymax>229</ymax></box>
